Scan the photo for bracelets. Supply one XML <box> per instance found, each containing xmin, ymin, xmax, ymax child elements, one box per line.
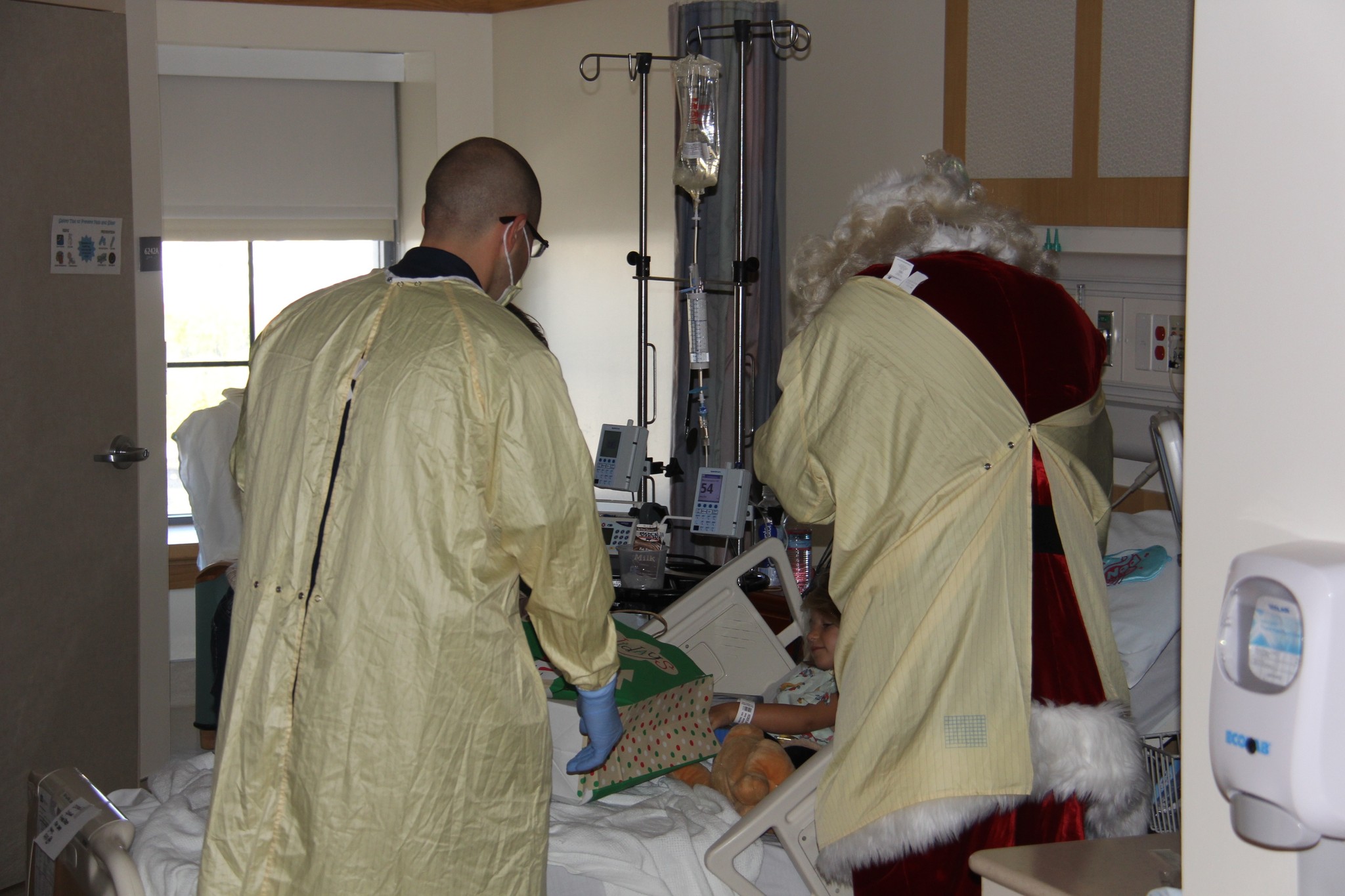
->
<box><xmin>731</xmin><ymin>695</ymin><xmax>756</xmax><ymax>725</ymax></box>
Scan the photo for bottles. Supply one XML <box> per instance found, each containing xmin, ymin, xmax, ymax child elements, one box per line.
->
<box><xmin>783</xmin><ymin>508</ymin><xmax>812</xmax><ymax>596</ymax></box>
<box><xmin>754</xmin><ymin>484</ymin><xmax>785</xmax><ymax>587</ymax></box>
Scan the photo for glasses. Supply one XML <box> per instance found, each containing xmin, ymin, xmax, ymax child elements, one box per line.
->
<box><xmin>499</xmin><ymin>215</ymin><xmax>549</xmax><ymax>258</ymax></box>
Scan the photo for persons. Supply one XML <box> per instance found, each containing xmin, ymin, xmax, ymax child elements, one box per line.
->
<box><xmin>168</xmin><ymin>384</ymin><xmax>254</xmax><ymax>753</ymax></box>
<box><xmin>729</xmin><ymin>151</ymin><xmax>1155</xmax><ymax>894</ymax></box>
<box><xmin>193</xmin><ymin>133</ymin><xmax>623</xmax><ymax>896</ymax></box>
<box><xmin>709</xmin><ymin>565</ymin><xmax>849</xmax><ymax>736</ymax></box>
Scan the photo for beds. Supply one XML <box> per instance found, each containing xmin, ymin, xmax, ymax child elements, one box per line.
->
<box><xmin>23</xmin><ymin>503</ymin><xmax>1185</xmax><ymax>896</ymax></box>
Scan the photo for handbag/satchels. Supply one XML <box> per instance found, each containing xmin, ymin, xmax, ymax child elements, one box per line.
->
<box><xmin>520</xmin><ymin>607</ymin><xmax>705</xmax><ymax>707</ymax></box>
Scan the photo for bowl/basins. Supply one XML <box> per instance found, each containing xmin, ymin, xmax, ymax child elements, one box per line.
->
<box><xmin>616</xmin><ymin>543</ymin><xmax>670</xmax><ymax>591</ymax></box>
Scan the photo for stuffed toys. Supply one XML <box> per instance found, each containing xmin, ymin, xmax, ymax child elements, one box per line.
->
<box><xmin>661</xmin><ymin>722</ymin><xmax>796</xmax><ymax>839</ymax></box>
